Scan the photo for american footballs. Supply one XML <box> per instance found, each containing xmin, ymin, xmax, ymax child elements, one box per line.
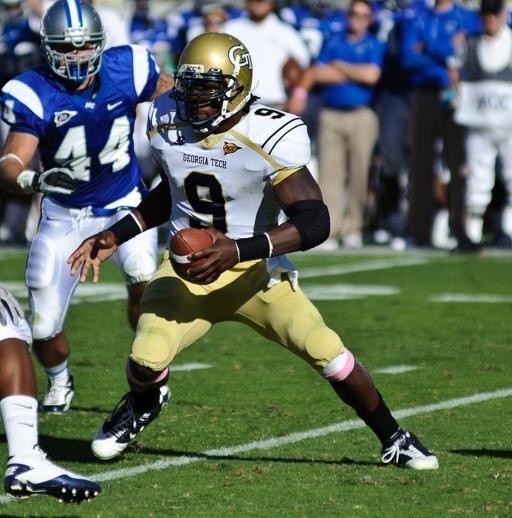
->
<box><xmin>169</xmin><ymin>227</ymin><xmax>219</xmax><ymax>285</ymax></box>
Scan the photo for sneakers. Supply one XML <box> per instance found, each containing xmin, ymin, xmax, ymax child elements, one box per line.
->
<box><xmin>90</xmin><ymin>385</ymin><xmax>171</xmax><ymax>460</ymax></box>
<box><xmin>3</xmin><ymin>455</ymin><xmax>103</xmax><ymax>507</ymax></box>
<box><xmin>381</xmin><ymin>431</ymin><xmax>440</xmax><ymax>471</ymax></box>
<box><xmin>38</xmin><ymin>375</ymin><xmax>76</xmax><ymax>416</ymax></box>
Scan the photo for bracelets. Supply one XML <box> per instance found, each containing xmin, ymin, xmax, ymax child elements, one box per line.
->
<box><xmin>236</xmin><ymin>233</ymin><xmax>274</xmax><ymax>263</ymax></box>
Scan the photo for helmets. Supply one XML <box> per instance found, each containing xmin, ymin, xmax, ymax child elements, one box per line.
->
<box><xmin>168</xmin><ymin>31</ymin><xmax>253</xmax><ymax>136</ymax></box>
<box><xmin>40</xmin><ymin>0</ymin><xmax>106</xmax><ymax>82</ymax></box>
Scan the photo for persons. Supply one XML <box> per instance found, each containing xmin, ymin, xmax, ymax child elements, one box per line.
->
<box><xmin>1</xmin><ymin>0</ymin><xmax>512</xmax><ymax>255</ymax></box>
<box><xmin>1</xmin><ymin>0</ymin><xmax>176</xmax><ymax>415</ymax></box>
<box><xmin>66</xmin><ymin>32</ymin><xmax>439</xmax><ymax>471</ymax></box>
<box><xmin>1</xmin><ymin>286</ymin><xmax>102</xmax><ymax>505</ymax></box>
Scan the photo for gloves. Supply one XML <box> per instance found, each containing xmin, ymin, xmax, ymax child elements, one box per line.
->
<box><xmin>31</xmin><ymin>166</ymin><xmax>78</xmax><ymax>196</ymax></box>
